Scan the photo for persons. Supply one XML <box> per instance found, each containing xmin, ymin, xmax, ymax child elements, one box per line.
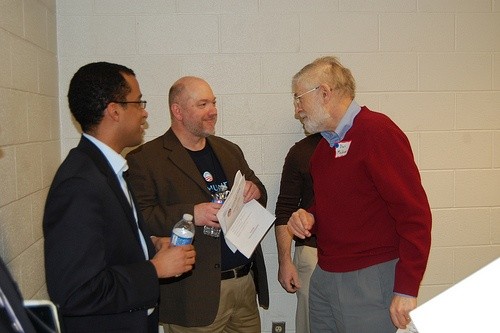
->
<box><xmin>274</xmin><ymin>102</ymin><xmax>323</xmax><ymax>333</ymax></box>
<box><xmin>43</xmin><ymin>62</ymin><xmax>196</xmax><ymax>333</ymax></box>
<box><xmin>122</xmin><ymin>76</ymin><xmax>269</xmax><ymax>333</ymax></box>
<box><xmin>287</xmin><ymin>56</ymin><xmax>432</xmax><ymax>333</ymax></box>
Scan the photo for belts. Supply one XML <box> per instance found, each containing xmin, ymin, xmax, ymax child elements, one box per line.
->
<box><xmin>221</xmin><ymin>263</ymin><xmax>251</xmax><ymax>280</ymax></box>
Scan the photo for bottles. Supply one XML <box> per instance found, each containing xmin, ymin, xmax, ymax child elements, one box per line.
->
<box><xmin>203</xmin><ymin>183</ymin><xmax>229</xmax><ymax>237</ymax></box>
<box><xmin>170</xmin><ymin>213</ymin><xmax>196</xmax><ymax>277</ymax></box>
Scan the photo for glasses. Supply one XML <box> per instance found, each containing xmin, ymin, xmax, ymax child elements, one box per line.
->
<box><xmin>293</xmin><ymin>86</ymin><xmax>332</xmax><ymax>103</ymax></box>
<box><xmin>103</xmin><ymin>100</ymin><xmax>146</xmax><ymax>110</ymax></box>
